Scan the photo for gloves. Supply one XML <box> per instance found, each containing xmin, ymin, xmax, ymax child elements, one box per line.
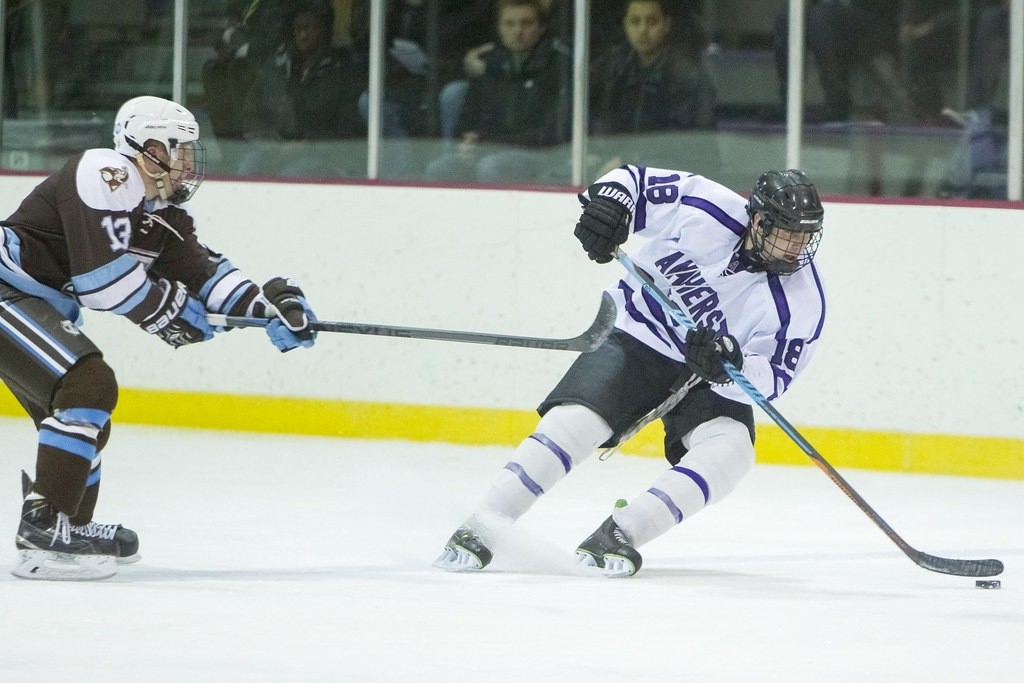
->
<box><xmin>574</xmin><ymin>182</ymin><xmax>636</xmax><ymax>264</ymax></box>
<box><xmin>683</xmin><ymin>327</ymin><xmax>743</xmax><ymax>384</ymax></box>
<box><xmin>237</xmin><ymin>276</ymin><xmax>318</xmax><ymax>353</ymax></box>
<box><xmin>139</xmin><ymin>278</ymin><xmax>214</xmax><ymax>350</ymax></box>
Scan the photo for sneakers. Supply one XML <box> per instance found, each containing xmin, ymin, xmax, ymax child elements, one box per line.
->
<box><xmin>53</xmin><ymin>513</ymin><xmax>142</xmax><ymax>565</ymax></box>
<box><xmin>9</xmin><ymin>469</ymin><xmax>119</xmax><ymax>581</ymax></box>
<box><xmin>567</xmin><ymin>514</ymin><xmax>642</xmax><ymax>578</ymax></box>
<box><xmin>417</xmin><ymin>517</ymin><xmax>494</xmax><ymax>572</ymax></box>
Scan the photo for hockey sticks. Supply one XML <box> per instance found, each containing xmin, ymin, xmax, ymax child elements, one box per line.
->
<box><xmin>205</xmin><ymin>291</ymin><xmax>616</xmax><ymax>355</ymax></box>
<box><xmin>577</xmin><ymin>191</ymin><xmax>1004</xmax><ymax>577</ymax></box>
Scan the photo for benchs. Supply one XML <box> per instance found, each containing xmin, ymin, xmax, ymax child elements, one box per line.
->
<box><xmin>718</xmin><ymin>123</ymin><xmax>1010</xmax><ymax>203</ymax></box>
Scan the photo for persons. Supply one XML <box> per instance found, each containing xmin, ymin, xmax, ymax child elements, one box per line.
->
<box><xmin>431</xmin><ymin>164</ymin><xmax>829</xmax><ymax>577</ymax></box>
<box><xmin>28</xmin><ymin>0</ymin><xmax>1007</xmax><ymax>188</ymax></box>
<box><xmin>0</xmin><ymin>96</ymin><xmax>317</xmax><ymax>581</ymax></box>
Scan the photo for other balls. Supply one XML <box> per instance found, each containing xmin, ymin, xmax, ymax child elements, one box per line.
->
<box><xmin>975</xmin><ymin>581</ymin><xmax>1002</xmax><ymax>590</ymax></box>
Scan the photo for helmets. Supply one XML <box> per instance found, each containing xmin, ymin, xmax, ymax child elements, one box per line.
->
<box><xmin>112</xmin><ymin>96</ymin><xmax>199</xmax><ymax>160</ymax></box>
<box><xmin>745</xmin><ymin>168</ymin><xmax>824</xmax><ymax>232</ymax></box>
